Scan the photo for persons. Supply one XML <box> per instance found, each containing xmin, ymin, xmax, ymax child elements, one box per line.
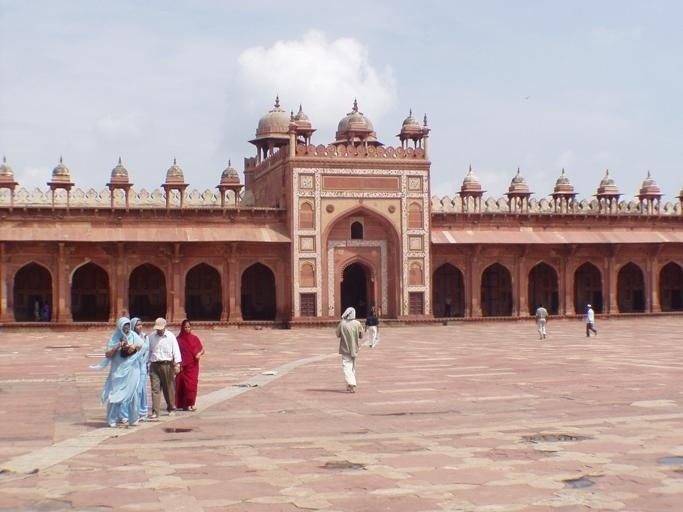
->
<box><xmin>118</xmin><ymin>317</ymin><xmax>155</xmax><ymax>423</ymax></box>
<box><xmin>174</xmin><ymin>320</ymin><xmax>204</xmax><ymax>411</ymax></box>
<box><xmin>535</xmin><ymin>303</ymin><xmax>548</xmax><ymax>340</ymax></box>
<box><xmin>586</xmin><ymin>303</ymin><xmax>597</xmax><ymax>337</ymax></box>
<box><xmin>33</xmin><ymin>296</ymin><xmax>50</xmax><ymax>322</ymax></box>
<box><xmin>146</xmin><ymin>317</ymin><xmax>181</xmax><ymax>420</ymax></box>
<box><xmin>442</xmin><ymin>293</ymin><xmax>452</xmax><ymax>317</ymax></box>
<box><xmin>89</xmin><ymin>316</ymin><xmax>143</xmax><ymax>429</ymax></box>
<box><xmin>335</xmin><ymin>307</ymin><xmax>364</xmax><ymax>393</ymax></box>
<box><xmin>364</xmin><ymin>310</ymin><xmax>380</xmax><ymax>349</ymax></box>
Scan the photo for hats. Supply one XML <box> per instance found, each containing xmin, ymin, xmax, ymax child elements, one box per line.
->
<box><xmin>153</xmin><ymin>318</ymin><xmax>166</xmax><ymax>330</ymax></box>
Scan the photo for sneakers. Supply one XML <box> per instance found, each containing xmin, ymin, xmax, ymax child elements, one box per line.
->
<box><xmin>169</xmin><ymin>411</ymin><xmax>175</xmax><ymax>416</ymax></box>
<box><xmin>151</xmin><ymin>411</ymin><xmax>157</xmax><ymax>418</ymax></box>
